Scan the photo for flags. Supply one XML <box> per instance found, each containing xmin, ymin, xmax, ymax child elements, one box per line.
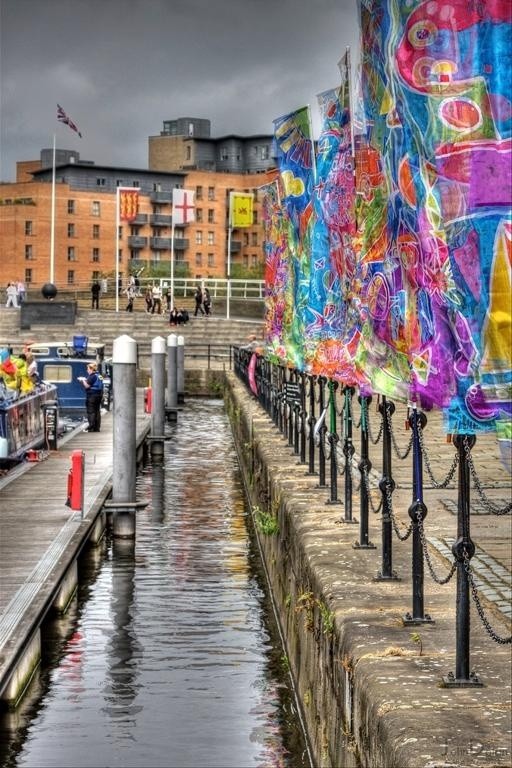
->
<box><xmin>172</xmin><ymin>188</ymin><xmax>196</xmax><ymax>226</ymax></box>
<box><xmin>230</xmin><ymin>192</ymin><xmax>253</xmax><ymax>230</ymax></box>
<box><xmin>56</xmin><ymin>102</ymin><xmax>83</xmax><ymax>138</ymax></box>
<box><xmin>118</xmin><ymin>186</ymin><xmax>139</xmax><ymax>222</ymax></box>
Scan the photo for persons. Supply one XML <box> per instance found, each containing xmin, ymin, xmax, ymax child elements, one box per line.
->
<box><xmin>1</xmin><ymin>351</ymin><xmax>40</xmax><ymax>405</ymax></box>
<box><xmin>91</xmin><ymin>279</ymin><xmax>100</xmax><ymax>310</ymax></box>
<box><xmin>117</xmin><ymin>270</ymin><xmax>214</xmax><ymax>327</ymax></box>
<box><xmin>6</xmin><ymin>281</ymin><xmax>21</xmax><ymax>309</ymax></box>
<box><xmin>16</xmin><ymin>281</ymin><xmax>26</xmax><ymax>301</ymax></box>
<box><xmin>78</xmin><ymin>363</ymin><xmax>104</xmax><ymax>435</ymax></box>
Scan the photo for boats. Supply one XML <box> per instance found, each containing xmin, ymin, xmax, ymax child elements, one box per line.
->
<box><xmin>0</xmin><ymin>336</ymin><xmax>105</xmax><ymax>460</ymax></box>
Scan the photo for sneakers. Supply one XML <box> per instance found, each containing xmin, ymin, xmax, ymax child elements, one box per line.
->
<box><xmin>82</xmin><ymin>426</ymin><xmax>101</xmax><ymax>433</ymax></box>
